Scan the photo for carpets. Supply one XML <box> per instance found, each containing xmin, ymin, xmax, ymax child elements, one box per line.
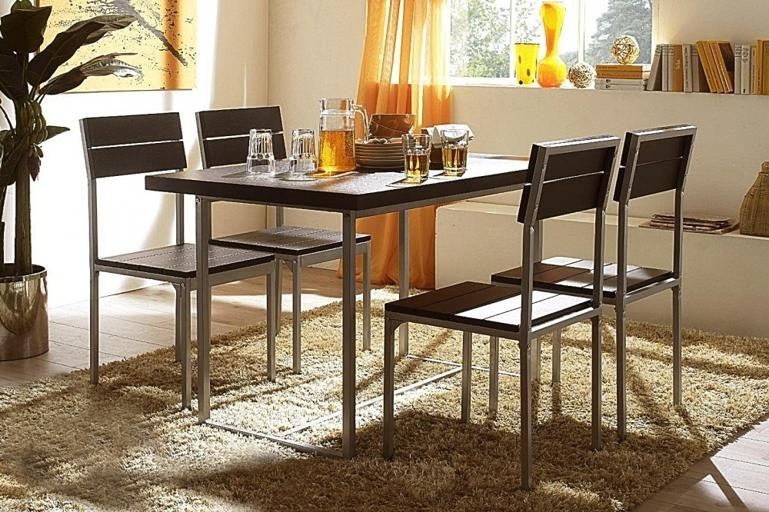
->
<box><xmin>1</xmin><ymin>286</ymin><xmax>769</xmax><ymax>512</ymax></box>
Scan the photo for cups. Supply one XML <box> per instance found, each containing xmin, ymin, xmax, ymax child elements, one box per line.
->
<box><xmin>514</xmin><ymin>42</ymin><xmax>539</xmax><ymax>85</ymax></box>
<box><xmin>402</xmin><ymin>134</ymin><xmax>430</xmax><ymax>181</ymax></box>
<box><xmin>246</xmin><ymin>128</ymin><xmax>275</xmax><ymax>173</ymax></box>
<box><xmin>440</xmin><ymin>129</ymin><xmax>468</xmax><ymax>176</ymax></box>
<box><xmin>288</xmin><ymin>128</ymin><xmax>316</xmax><ymax>174</ymax></box>
<box><xmin>317</xmin><ymin>97</ymin><xmax>369</xmax><ymax>174</ymax></box>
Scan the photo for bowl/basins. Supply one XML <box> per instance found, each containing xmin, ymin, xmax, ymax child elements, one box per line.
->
<box><xmin>369</xmin><ymin>113</ymin><xmax>416</xmax><ymax>137</ymax></box>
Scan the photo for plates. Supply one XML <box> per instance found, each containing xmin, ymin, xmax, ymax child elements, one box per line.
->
<box><xmin>354</xmin><ymin>138</ymin><xmax>406</xmax><ymax>169</ymax></box>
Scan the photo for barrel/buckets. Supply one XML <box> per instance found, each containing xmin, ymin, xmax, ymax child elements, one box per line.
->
<box><xmin>1</xmin><ymin>263</ymin><xmax>50</xmax><ymax>362</ymax></box>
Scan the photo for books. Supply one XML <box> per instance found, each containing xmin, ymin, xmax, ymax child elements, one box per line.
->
<box><xmin>639</xmin><ymin>211</ymin><xmax>740</xmax><ymax>234</ymax></box>
<box><xmin>594</xmin><ymin>39</ymin><xmax>769</xmax><ymax>95</ymax></box>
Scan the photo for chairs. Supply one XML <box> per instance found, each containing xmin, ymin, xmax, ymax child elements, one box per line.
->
<box><xmin>194</xmin><ymin>105</ymin><xmax>372</xmax><ymax>372</ymax></box>
<box><xmin>78</xmin><ymin>111</ymin><xmax>277</xmax><ymax>409</ymax></box>
<box><xmin>384</xmin><ymin>133</ymin><xmax>621</xmax><ymax>491</ymax></box>
<box><xmin>489</xmin><ymin>122</ymin><xmax>699</xmax><ymax>439</ymax></box>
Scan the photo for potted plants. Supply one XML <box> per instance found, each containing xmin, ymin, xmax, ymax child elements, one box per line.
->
<box><xmin>0</xmin><ymin>1</ymin><xmax>141</xmax><ymax>362</ymax></box>
